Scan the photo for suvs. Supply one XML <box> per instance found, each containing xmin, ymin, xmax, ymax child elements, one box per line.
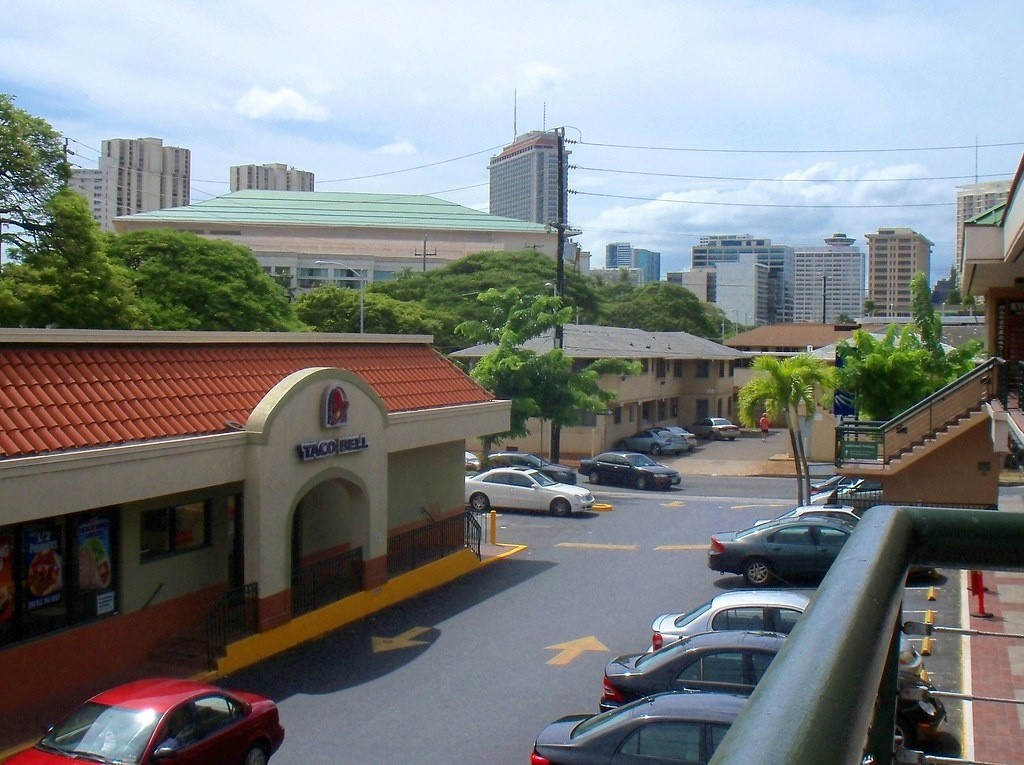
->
<box><xmin>479</xmin><ymin>450</ymin><xmax>576</xmax><ymax>485</ymax></box>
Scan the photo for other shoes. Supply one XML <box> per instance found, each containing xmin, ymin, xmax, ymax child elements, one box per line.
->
<box><xmin>762</xmin><ymin>439</ymin><xmax>766</xmax><ymax>442</ymax></box>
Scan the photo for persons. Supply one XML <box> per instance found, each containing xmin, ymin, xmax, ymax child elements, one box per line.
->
<box><xmin>760</xmin><ymin>413</ymin><xmax>772</xmax><ymax>444</ymax></box>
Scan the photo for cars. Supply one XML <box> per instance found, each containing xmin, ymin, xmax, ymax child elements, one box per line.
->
<box><xmin>617</xmin><ymin>428</ymin><xmax>690</xmax><ymax>456</ymax></box>
<box><xmin>653</xmin><ymin>425</ymin><xmax>697</xmax><ymax>452</ymax></box>
<box><xmin>577</xmin><ymin>450</ymin><xmax>681</xmax><ymax>491</ymax></box>
<box><xmin>754</xmin><ymin>503</ymin><xmax>864</xmax><ymax>545</ymax></box>
<box><xmin>810</xmin><ymin>474</ymin><xmax>882</xmax><ymax>496</ymax></box>
<box><xmin>599</xmin><ymin>628</ymin><xmax>946</xmax><ymax>749</ymax></box>
<box><xmin>465</xmin><ymin>465</ymin><xmax>596</xmax><ymax>518</ymax></box>
<box><xmin>646</xmin><ymin>587</ymin><xmax>924</xmax><ymax>678</ymax></box>
<box><xmin>0</xmin><ymin>677</ymin><xmax>286</xmax><ymax>765</ymax></box>
<box><xmin>686</xmin><ymin>417</ymin><xmax>740</xmax><ymax>442</ymax></box>
<box><xmin>707</xmin><ymin>517</ymin><xmax>935</xmax><ymax>590</ymax></box>
<box><xmin>464</xmin><ymin>450</ymin><xmax>480</xmax><ymax>472</ymax></box>
<box><xmin>530</xmin><ymin>690</ymin><xmax>906</xmax><ymax>765</ymax></box>
